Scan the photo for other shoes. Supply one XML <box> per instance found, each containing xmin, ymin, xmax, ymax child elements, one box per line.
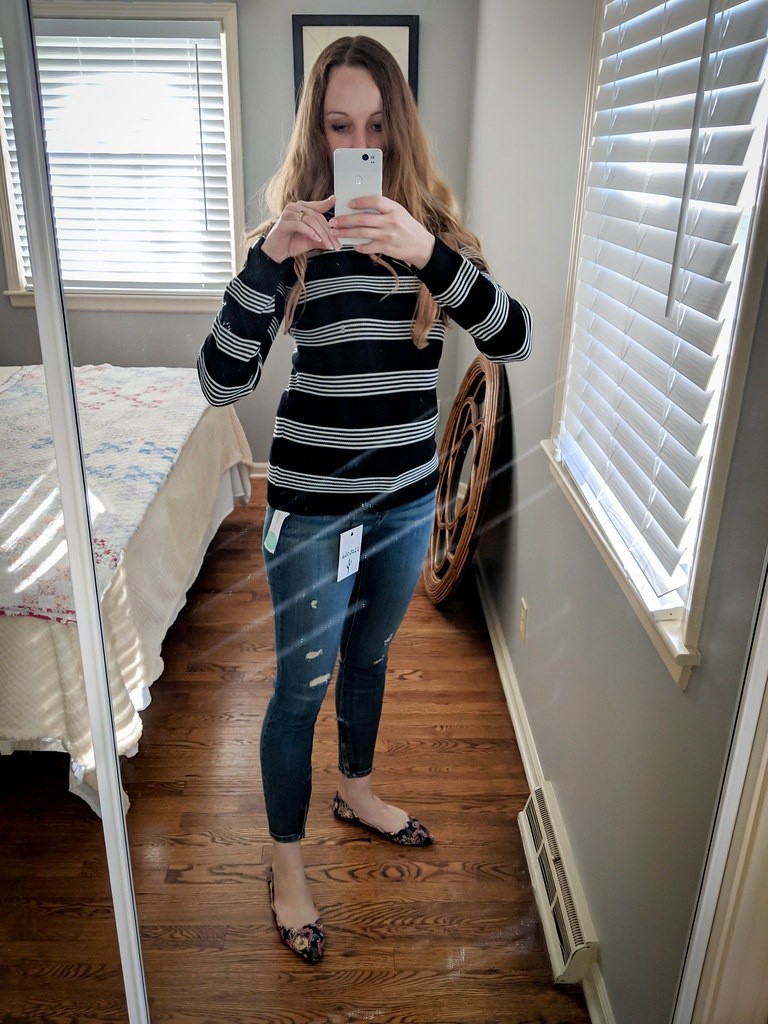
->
<box><xmin>332</xmin><ymin>789</ymin><xmax>435</xmax><ymax>847</ymax></box>
<box><xmin>268</xmin><ymin>863</ymin><xmax>325</xmax><ymax>963</ymax></box>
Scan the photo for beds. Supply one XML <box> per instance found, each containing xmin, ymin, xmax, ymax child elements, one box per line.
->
<box><xmin>0</xmin><ymin>364</ymin><xmax>253</xmax><ymax>823</ymax></box>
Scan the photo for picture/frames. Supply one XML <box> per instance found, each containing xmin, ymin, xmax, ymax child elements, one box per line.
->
<box><xmin>291</xmin><ymin>13</ymin><xmax>419</xmax><ymax>124</ymax></box>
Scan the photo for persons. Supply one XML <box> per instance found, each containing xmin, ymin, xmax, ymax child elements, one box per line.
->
<box><xmin>194</xmin><ymin>36</ymin><xmax>532</xmax><ymax>966</ymax></box>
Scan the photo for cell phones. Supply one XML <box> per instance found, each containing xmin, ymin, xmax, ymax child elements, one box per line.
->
<box><xmin>333</xmin><ymin>148</ymin><xmax>383</xmax><ymax>245</ymax></box>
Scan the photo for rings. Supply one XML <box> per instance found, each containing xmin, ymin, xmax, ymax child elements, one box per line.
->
<box><xmin>299</xmin><ymin>210</ymin><xmax>305</xmax><ymax>221</ymax></box>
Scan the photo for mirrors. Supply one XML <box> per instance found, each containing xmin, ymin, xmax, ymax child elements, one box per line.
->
<box><xmin>0</xmin><ymin>0</ymin><xmax>768</xmax><ymax>1024</ymax></box>
